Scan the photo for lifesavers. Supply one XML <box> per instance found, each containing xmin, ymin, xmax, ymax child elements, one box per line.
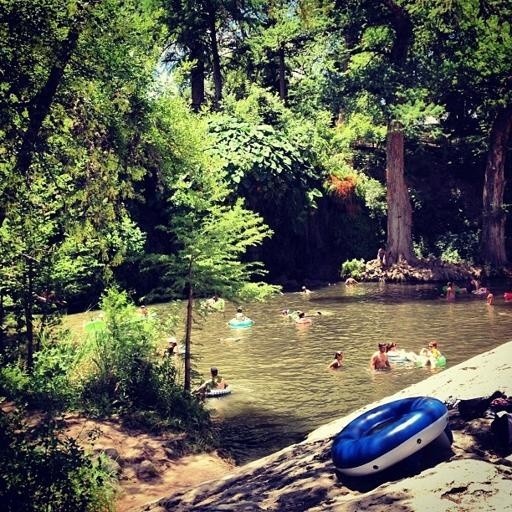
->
<box><xmin>331</xmin><ymin>396</ymin><xmax>453</xmax><ymax>481</ymax></box>
<box><xmin>375</xmin><ymin>351</ymin><xmax>447</xmax><ymax>368</ymax></box>
<box><xmin>200</xmin><ymin>388</ymin><xmax>231</xmax><ymax>398</ymax></box>
<box><xmin>228</xmin><ymin>319</ymin><xmax>256</xmax><ymax>328</ymax></box>
<box><xmin>201</xmin><ymin>297</ymin><xmax>225</xmax><ymax>311</ymax></box>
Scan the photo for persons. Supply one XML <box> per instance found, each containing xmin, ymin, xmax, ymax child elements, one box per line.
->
<box><xmin>445</xmin><ymin>276</ymin><xmax>494</xmax><ymax>306</ymax></box>
<box><xmin>192</xmin><ymin>367</ymin><xmax>227</xmax><ymax>393</ymax></box>
<box><xmin>141</xmin><ymin>304</ymin><xmax>149</xmax><ymax>317</ymax></box>
<box><xmin>329</xmin><ymin>351</ymin><xmax>342</xmax><ymax>369</ymax></box>
<box><xmin>370</xmin><ymin>338</ymin><xmax>441</xmax><ymax>370</ymax></box>
<box><xmin>377</xmin><ymin>246</ymin><xmax>388</xmax><ymax>264</ymax></box>
<box><xmin>165</xmin><ymin>338</ymin><xmax>179</xmax><ymax>355</ymax></box>
<box><xmin>206</xmin><ymin>295</ymin><xmax>218</xmax><ymax>308</ymax></box>
<box><xmin>345</xmin><ymin>274</ymin><xmax>358</xmax><ymax>286</ymax></box>
<box><xmin>504</xmin><ymin>290</ymin><xmax>512</xmax><ymax>304</ymax></box>
<box><xmin>280</xmin><ymin>308</ymin><xmax>321</xmax><ymax>323</ymax></box>
<box><xmin>237</xmin><ymin>308</ymin><xmax>244</xmax><ymax>320</ymax></box>
<box><xmin>301</xmin><ymin>286</ymin><xmax>310</xmax><ymax>294</ymax></box>
